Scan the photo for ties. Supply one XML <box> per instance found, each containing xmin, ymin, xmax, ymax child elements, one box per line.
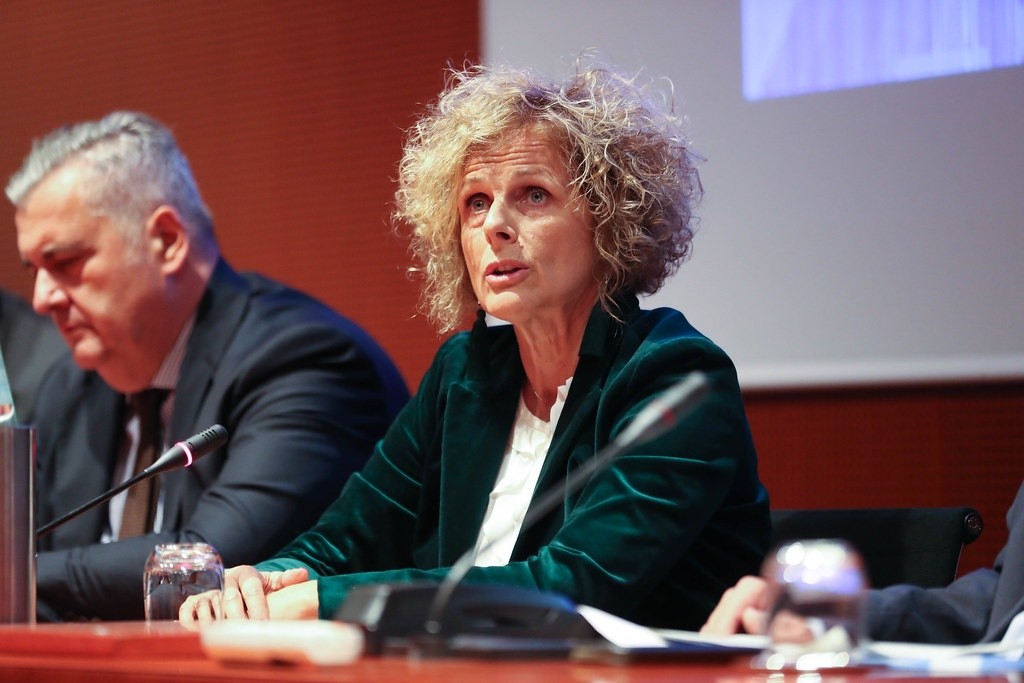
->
<box><xmin>117</xmin><ymin>405</ymin><xmax>163</xmax><ymax>540</ymax></box>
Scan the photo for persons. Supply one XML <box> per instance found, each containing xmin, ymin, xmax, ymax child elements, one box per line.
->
<box><xmin>178</xmin><ymin>54</ymin><xmax>772</xmax><ymax>633</ymax></box>
<box><xmin>700</xmin><ymin>482</ymin><xmax>1024</xmax><ymax>646</ymax></box>
<box><xmin>0</xmin><ymin>111</ymin><xmax>412</xmax><ymax>623</ymax></box>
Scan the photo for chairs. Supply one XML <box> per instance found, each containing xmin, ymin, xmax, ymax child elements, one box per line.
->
<box><xmin>770</xmin><ymin>505</ymin><xmax>985</xmax><ymax>591</ymax></box>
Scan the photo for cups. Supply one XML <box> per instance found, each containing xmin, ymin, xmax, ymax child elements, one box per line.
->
<box><xmin>761</xmin><ymin>533</ymin><xmax>869</xmax><ymax>670</ymax></box>
<box><xmin>142</xmin><ymin>539</ymin><xmax>227</xmax><ymax>634</ymax></box>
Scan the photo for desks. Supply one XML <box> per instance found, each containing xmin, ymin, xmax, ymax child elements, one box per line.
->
<box><xmin>0</xmin><ymin>621</ymin><xmax>1024</xmax><ymax>683</ymax></box>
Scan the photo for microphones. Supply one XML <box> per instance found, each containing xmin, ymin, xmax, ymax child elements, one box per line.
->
<box><xmin>34</xmin><ymin>423</ymin><xmax>229</xmax><ymax>540</ymax></box>
